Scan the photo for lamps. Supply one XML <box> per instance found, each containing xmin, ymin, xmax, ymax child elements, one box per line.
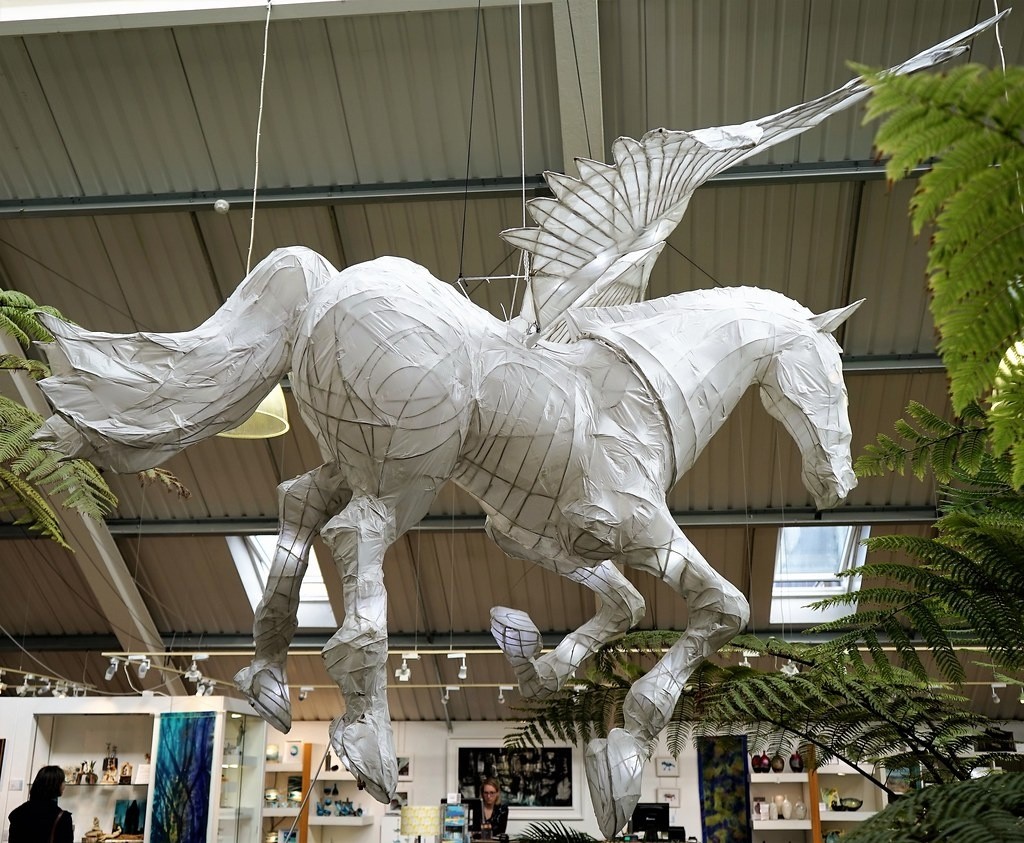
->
<box><xmin>216</xmin><ymin>0</ymin><xmax>290</xmax><ymax>438</ymax></box>
<box><xmin>498</xmin><ymin>687</ymin><xmax>514</xmax><ymax>703</ymax></box>
<box><xmin>739</xmin><ymin>651</ymin><xmax>760</xmax><ymax>667</ymax></box>
<box><xmin>441</xmin><ymin>687</ymin><xmax>460</xmax><ymax>704</ymax></box>
<box><xmin>447</xmin><ymin>653</ymin><xmax>467</xmax><ymax>679</ymax></box>
<box><xmin>0</xmin><ymin>655</ymin><xmax>215</xmax><ymax>698</ymax></box>
<box><xmin>298</xmin><ymin>687</ymin><xmax>314</xmax><ymax>700</ymax></box>
<box><xmin>395</xmin><ymin>653</ymin><xmax>421</xmax><ymax>681</ymax></box>
<box><xmin>400</xmin><ymin>806</ymin><xmax>440</xmax><ymax>843</ymax></box>
<box><xmin>991</xmin><ymin>683</ymin><xmax>1024</xmax><ymax>703</ymax></box>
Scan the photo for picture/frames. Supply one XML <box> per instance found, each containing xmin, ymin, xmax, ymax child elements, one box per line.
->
<box><xmin>385</xmin><ymin>788</ymin><xmax>413</xmax><ymax>813</ymax></box>
<box><xmin>655</xmin><ymin>757</ymin><xmax>681</xmax><ymax>807</ymax></box>
<box><xmin>447</xmin><ymin>739</ymin><xmax>584</xmax><ymax>821</ymax></box>
<box><xmin>396</xmin><ymin>753</ymin><xmax>414</xmax><ymax>782</ymax></box>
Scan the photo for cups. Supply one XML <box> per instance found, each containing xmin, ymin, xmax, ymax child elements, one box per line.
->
<box><xmin>760</xmin><ymin>803</ymin><xmax>769</xmax><ymax>820</ymax></box>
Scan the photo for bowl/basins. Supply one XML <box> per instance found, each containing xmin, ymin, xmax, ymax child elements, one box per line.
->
<box><xmin>840</xmin><ymin>796</ymin><xmax>863</xmax><ymax>811</ymax></box>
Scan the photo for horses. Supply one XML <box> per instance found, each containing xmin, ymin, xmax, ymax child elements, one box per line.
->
<box><xmin>31</xmin><ymin>248</ymin><xmax>869</xmax><ymax>841</ymax></box>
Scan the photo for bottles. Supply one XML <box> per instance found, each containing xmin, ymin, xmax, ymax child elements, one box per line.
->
<box><xmin>751</xmin><ymin>755</ymin><xmax>761</xmax><ymax>773</ymax></box>
<box><xmin>759</xmin><ymin>750</ymin><xmax>771</xmax><ymax>773</ymax></box>
<box><xmin>790</xmin><ymin>751</ymin><xmax>804</xmax><ymax>773</ymax></box>
<box><xmin>769</xmin><ymin>796</ymin><xmax>777</xmax><ymax>820</ymax></box>
<box><xmin>795</xmin><ymin>796</ymin><xmax>806</xmax><ymax>819</ymax></box>
<box><xmin>771</xmin><ymin>751</ymin><xmax>785</xmax><ymax>773</ymax></box>
<box><xmin>781</xmin><ymin>794</ymin><xmax>793</xmax><ymax>819</ymax></box>
<box><xmin>775</xmin><ymin>795</ymin><xmax>785</xmax><ymax>819</ymax></box>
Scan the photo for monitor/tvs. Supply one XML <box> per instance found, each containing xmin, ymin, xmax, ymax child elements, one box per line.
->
<box><xmin>440</xmin><ymin>798</ymin><xmax>482</xmax><ymax>832</ymax></box>
<box><xmin>630</xmin><ymin>801</ymin><xmax>669</xmax><ymax>842</ymax></box>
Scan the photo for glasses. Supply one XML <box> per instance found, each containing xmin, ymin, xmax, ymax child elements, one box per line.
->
<box><xmin>483</xmin><ymin>791</ymin><xmax>497</xmax><ymax>795</ymax></box>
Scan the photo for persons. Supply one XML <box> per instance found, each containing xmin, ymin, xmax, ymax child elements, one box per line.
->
<box><xmin>8</xmin><ymin>766</ymin><xmax>74</xmax><ymax>843</ymax></box>
<box><xmin>473</xmin><ymin>779</ymin><xmax>508</xmax><ymax>838</ymax></box>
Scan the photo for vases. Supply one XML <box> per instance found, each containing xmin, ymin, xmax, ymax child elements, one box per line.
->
<box><xmin>752</xmin><ymin>750</ymin><xmax>807</xmax><ymax>819</ymax></box>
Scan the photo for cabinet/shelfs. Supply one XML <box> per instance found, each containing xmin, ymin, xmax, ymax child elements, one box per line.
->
<box><xmin>751</xmin><ymin>773</ymin><xmax>812</xmax><ymax>830</ymax></box>
<box><xmin>309</xmin><ymin>771</ymin><xmax>375</xmax><ymax>826</ymax></box>
<box><xmin>817</xmin><ymin>763</ymin><xmax>879</xmax><ymax>821</ymax></box>
<box><xmin>262</xmin><ymin>763</ymin><xmax>303</xmax><ymax>817</ymax></box>
<box><xmin>219</xmin><ymin>755</ymin><xmax>258</xmax><ymax>821</ymax></box>
